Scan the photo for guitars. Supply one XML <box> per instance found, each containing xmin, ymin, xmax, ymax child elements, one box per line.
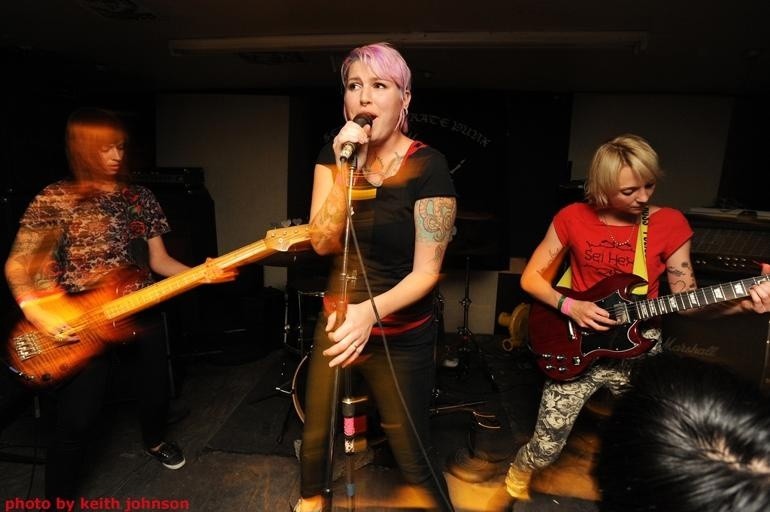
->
<box><xmin>9</xmin><ymin>220</ymin><xmax>310</xmax><ymax>383</ymax></box>
<box><xmin>528</xmin><ymin>273</ymin><xmax>769</xmax><ymax>383</ymax></box>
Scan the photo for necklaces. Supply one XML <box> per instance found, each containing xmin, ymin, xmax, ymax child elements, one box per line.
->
<box><xmin>602</xmin><ymin>212</ymin><xmax>637</xmax><ymax>246</ymax></box>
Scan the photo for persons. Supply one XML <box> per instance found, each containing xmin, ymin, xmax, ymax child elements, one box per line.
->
<box><xmin>505</xmin><ymin>135</ymin><xmax>770</xmax><ymax>502</ymax></box>
<box><xmin>295</xmin><ymin>41</ymin><xmax>458</xmax><ymax>512</ymax></box>
<box><xmin>5</xmin><ymin>110</ymin><xmax>239</xmax><ymax>471</ymax></box>
<box><xmin>590</xmin><ymin>353</ymin><xmax>770</xmax><ymax>511</ymax></box>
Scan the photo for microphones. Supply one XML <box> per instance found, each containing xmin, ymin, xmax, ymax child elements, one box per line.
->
<box><xmin>340</xmin><ymin>113</ymin><xmax>372</xmax><ymax>161</ymax></box>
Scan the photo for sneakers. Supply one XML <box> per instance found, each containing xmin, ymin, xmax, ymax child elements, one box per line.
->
<box><xmin>292</xmin><ymin>492</ymin><xmax>325</xmax><ymax>512</ymax></box>
<box><xmin>504</xmin><ymin>461</ymin><xmax>532</xmax><ymax>499</ymax></box>
<box><xmin>140</xmin><ymin>438</ymin><xmax>186</xmax><ymax>469</ymax></box>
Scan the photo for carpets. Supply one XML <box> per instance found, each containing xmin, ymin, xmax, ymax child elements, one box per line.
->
<box><xmin>200</xmin><ymin>337</ymin><xmax>517</xmax><ymax>464</ymax></box>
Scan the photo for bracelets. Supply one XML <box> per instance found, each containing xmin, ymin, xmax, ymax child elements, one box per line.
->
<box><xmin>557</xmin><ymin>294</ymin><xmax>568</xmax><ymax>311</ymax></box>
<box><xmin>561</xmin><ymin>296</ymin><xmax>572</xmax><ymax>315</ymax></box>
<box><xmin>16</xmin><ymin>292</ymin><xmax>36</xmax><ymax>308</ymax></box>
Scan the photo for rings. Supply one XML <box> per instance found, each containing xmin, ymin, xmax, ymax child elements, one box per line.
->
<box><xmin>356</xmin><ymin>348</ymin><xmax>362</xmax><ymax>354</ymax></box>
<box><xmin>353</xmin><ymin>342</ymin><xmax>358</xmax><ymax>348</ymax></box>
<box><xmin>53</xmin><ymin>333</ymin><xmax>66</xmax><ymax>342</ymax></box>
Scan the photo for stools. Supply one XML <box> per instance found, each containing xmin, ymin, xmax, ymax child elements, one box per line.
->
<box><xmin>278</xmin><ymin>276</ymin><xmax>325</xmax><ymax>356</ymax></box>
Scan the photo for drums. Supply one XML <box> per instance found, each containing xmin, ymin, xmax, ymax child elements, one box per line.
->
<box><xmin>284</xmin><ymin>276</ymin><xmax>328</xmax><ymax>348</ymax></box>
<box><xmin>292</xmin><ymin>353</ymin><xmax>387</xmax><ymax>452</ymax></box>
